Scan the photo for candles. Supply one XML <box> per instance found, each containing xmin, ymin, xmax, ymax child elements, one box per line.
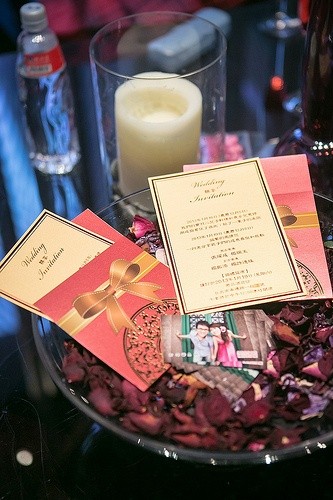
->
<box><xmin>115</xmin><ymin>72</ymin><xmax>202</xmax><ymax>214</ymax></box>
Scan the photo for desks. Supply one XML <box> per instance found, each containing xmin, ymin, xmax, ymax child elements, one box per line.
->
<box><xmin>0</xmin><ymin>26</ymin><xmax>333</xmax><ymax>500</ymax></box>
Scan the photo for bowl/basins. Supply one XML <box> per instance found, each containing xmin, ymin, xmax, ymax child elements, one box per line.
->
<box><xmin>30</xmin><ymin>188</ymin><xmax>332</xmax><ymax>468</ymax></box>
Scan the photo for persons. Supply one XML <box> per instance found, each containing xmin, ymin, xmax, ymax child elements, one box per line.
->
<box><xmin>210</xmin><ymin>323</ymin><xmax>248</xmax><ymax>367</ymax></box>
<box><xmin>176</xmin><ymin>321</ymin><xmax>214</xmax><ymax>365</ymax></box>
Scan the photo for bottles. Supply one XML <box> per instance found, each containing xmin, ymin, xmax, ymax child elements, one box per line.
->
<box><xmin>271</xmin><ymin>1</ymin><xmax>332</xmax><ymax>195</ymax></box>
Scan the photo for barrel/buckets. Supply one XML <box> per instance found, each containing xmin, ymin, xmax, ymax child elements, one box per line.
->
<box><xmin>13</xmin><ymin>2</ymin><xmax>82</xmax><ymax>175</ymax></box>
